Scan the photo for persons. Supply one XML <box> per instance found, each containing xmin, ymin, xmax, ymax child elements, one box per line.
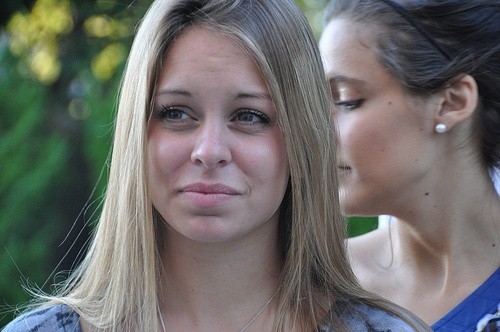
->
<box><xmin>0</xmin><ymin>1</ymin><xmax>428</xmax><ymax>331</ymax></box>
<box><xmin>312</xmin><ymin>1</ymin><xmax>499</xmax><ymax>330</ymax></box>
